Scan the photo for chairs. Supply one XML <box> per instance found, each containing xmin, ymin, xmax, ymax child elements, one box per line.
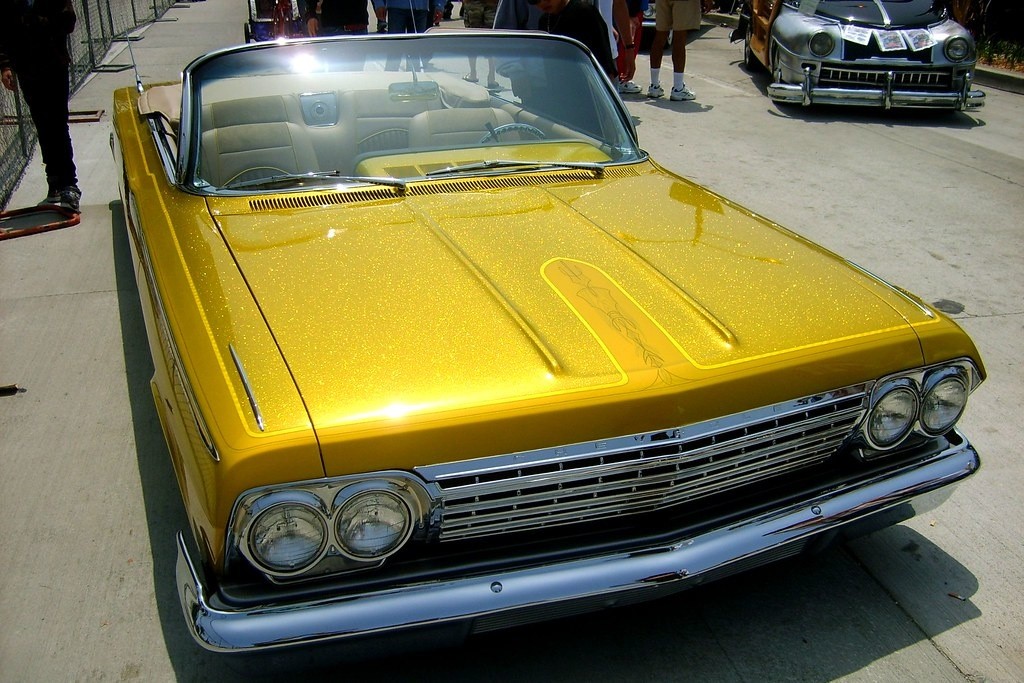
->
<box><xmin>201</xmin><ymin>121</ymin><xmax>322</xmax><ymax>187</ymax></box>
<box><xmin>409</xmin><ymin>107</ymin><xmax>520</xmax><ymax>148</ymax></box>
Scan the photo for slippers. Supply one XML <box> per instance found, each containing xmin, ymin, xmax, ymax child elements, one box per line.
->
<box><xmin>463</xmin><ymin>74</ymin><xmax>479</xmax><ymax>82</ymax></box>
<box><xmin>488</xmin><ymin>80</ymin><xmax>499</xmax><ymax>89</ymax></box>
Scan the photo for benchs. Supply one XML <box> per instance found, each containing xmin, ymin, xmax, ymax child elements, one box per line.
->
<box><xmin>202</xmin><ymin>87</ymin><xmax>443</xmax><ymax>182</ymax></box>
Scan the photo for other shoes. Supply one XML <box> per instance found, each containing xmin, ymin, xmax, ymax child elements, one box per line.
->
<box><xmin>423</xmin><ymin>62</ymin><xmax>433</xmax><ymax>72</ymax></box>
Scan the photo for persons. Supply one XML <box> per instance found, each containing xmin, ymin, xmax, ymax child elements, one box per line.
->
<box><xmin>0</xmin><ymin>0</ymin><xmax>82</xmax><ymax>212</ymax></box>
<box><xmin>647</xmin><ymin>0</ymin><xmax>713</xmax><ymax>100</ymax></box>
<box><xmin>296</xmin><ymin>0</ymin><xmax>643</xmax><ymax>138</ymax></box>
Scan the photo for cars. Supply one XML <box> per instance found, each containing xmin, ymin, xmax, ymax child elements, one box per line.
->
<box><xmin>729</xmin><ymin>0</ymin><xmax>986</xmax><ymax>114</ymax></box>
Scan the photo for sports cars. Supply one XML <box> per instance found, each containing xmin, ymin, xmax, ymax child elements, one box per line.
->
<box><xmin>111</xmin><ymin>25</ymin><xmax>987</xmax><ymax>655</ymax></box>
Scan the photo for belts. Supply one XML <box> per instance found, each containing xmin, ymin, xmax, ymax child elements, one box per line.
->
<box><xmin>343</xmin><ymin>25</ymin><xmax>367</xmax><ymax>31</ymax></box>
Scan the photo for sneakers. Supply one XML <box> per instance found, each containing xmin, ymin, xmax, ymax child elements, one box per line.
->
<box><xmin>647</xmin><ymin>84</ymin><xmax>664</xmax><ymax>97</ymax></box>
<box><xmin>37</xmin><ymin>196</ymin><xmax>62</xmax><ymax>207</ymax></box>
<box><xmin>617</xmin><ymin>80</ymin><xmax>643</xmax><ymax>93</ymax></box>
<box><xmin>59</xmin><ymin>186</ymin><xmax>81</xmax><ymax>212</ymax></box>
<box><xmin>670</xmin><ymin>82</ymin><xmax>696</xmax><ymax>101</ymax></box>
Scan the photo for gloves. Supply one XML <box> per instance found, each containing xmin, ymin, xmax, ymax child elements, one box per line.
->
<box><xmin>375</xmin><ymin>7</ymin><xmax>386</xmax><ymax>22</ymax></box>
<box><xmin>433</xmin><ymin>9</ymin><xmax>443</xmax><ymax>23</ymax></box>
